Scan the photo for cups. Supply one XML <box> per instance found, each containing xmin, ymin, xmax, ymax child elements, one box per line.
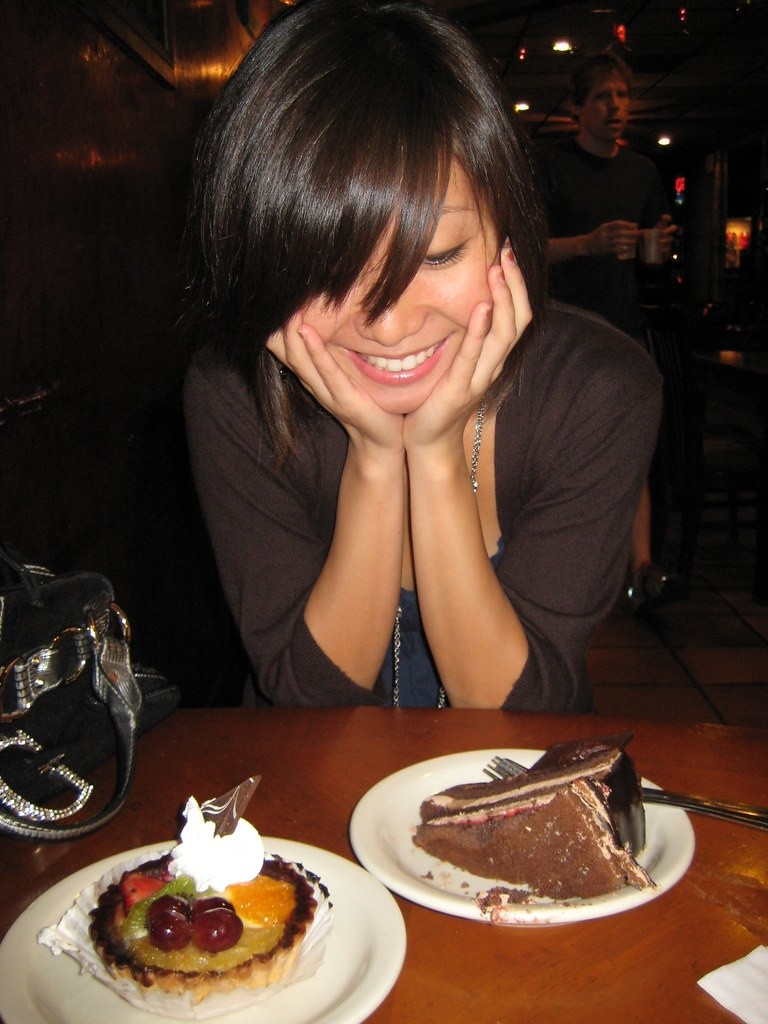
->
<box><xmin>640</xmin><ymin>229</ymin><xmax>668</xmax><ymax>263</ymax></box>
<box><xmin>611</xmin><ymin>226</ymin><xmax>635</xmax><ymax>260</ymax></box>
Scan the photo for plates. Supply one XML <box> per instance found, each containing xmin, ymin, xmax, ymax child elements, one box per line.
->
<box><xmin>0</xmin><ymin>834</ymin><xmax>408</xmax><ymax>1024</ymax></box>
<box><xmin>349</xmin><ymin>748</ymin><xmax>697</xmax><ymax>928</ymax></box>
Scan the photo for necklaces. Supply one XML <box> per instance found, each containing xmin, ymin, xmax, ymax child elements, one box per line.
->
<box><xmin>392</xmin><ymin>394</ymin><xmax>486</xmax><ymax>707</ymax></box>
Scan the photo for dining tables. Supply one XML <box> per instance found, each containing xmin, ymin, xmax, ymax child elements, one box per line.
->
<box><xmin>0</xmin><ymin>706</ymin><xmax>768</xmax><ymax>1024</ymax></box>
<box><xmin>685</xmin><ymin>349</ymin><xmax>768</xmax><ymax>605</ymax></box>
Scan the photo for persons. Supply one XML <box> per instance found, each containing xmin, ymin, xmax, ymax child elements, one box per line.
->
<box><xmin>538</xmin><ymin>52</ymin><xmax>677</xmax><ymax>623</ymax></box>
<box><xmin>182</xmin><ymin>0</ymin><xmax>662</xmax><ymax>712</ymax></box>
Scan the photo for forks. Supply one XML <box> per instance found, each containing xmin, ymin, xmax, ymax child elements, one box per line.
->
<box><xmin>483</xmin><ymin>756</ymin><xmax>768</xmax><ymax>831</ymax></box>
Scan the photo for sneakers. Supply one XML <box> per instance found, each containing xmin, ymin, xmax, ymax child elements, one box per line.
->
<box><xmin>631</xmin><ymin>565</ymin><xmax>692</xmax><ymax>611</ymax></box>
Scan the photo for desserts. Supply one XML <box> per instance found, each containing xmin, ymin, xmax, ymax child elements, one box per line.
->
<box><xmin>411</xmin><ymin>750</ymin><xmax>656</xmax><ymax>898</ymax></box>
<box><xmin>88</xmin><ymin>796</ymin><xmax>317</xmax><ymax>1000</ymax></box>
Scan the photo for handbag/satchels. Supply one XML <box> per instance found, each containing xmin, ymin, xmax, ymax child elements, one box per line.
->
<box><xmin>1</xmin><ymin>559</ymin><xmax>184</xmax><ymax>838</ymax></box>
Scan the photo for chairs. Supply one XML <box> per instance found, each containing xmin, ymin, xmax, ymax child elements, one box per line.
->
<box><xmin>635</xmin><ymin>299</ymin><xmax>768</xmax><ymax>577</ymax></box>
